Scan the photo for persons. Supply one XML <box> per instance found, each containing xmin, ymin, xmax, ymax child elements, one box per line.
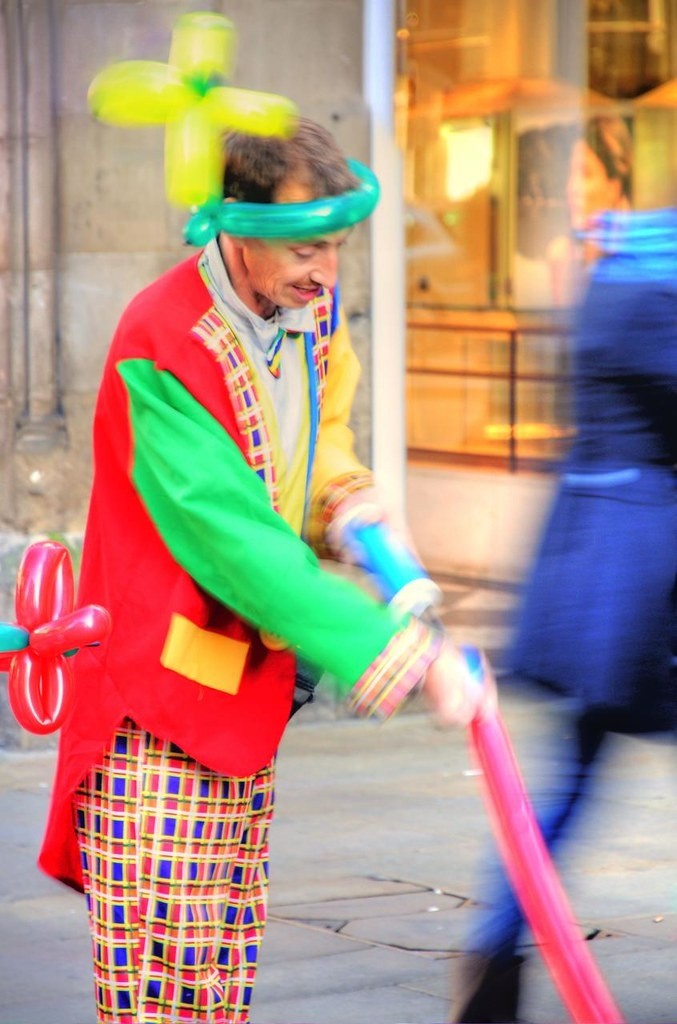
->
<box><xmin>453</xmin><ymin>195</ymin><xmax>677</xmax><ymax>1022</ymax></box>
<box><xmin>39</xmin><ymin>91</ymin><xmax>499</xmax><ymax>1022</ymax></box>
<box><xmin>539</xmin><ymin>115</ymin><xmax>635</xmax><ymax>305</ymax></box>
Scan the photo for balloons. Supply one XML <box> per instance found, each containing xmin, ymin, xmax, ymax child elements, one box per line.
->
<box><xmin>0</xmin><ymin>539</ymin><xmax>106</xmax><ymax>738</ymax></box>
<box><xmin>86</xmin><ymin>9</ymin><xmax>381</xmax><ymax>251</ymax></box>
<box><xmin>448</xmin><ymin>639</ymin><xmax>624</xmax><ymax>1024</ymax></box>
